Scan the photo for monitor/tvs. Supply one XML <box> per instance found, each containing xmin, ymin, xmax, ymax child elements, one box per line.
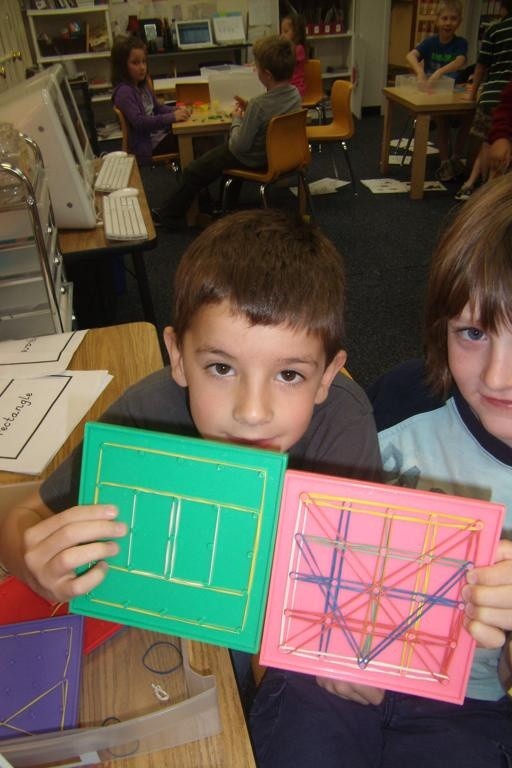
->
<box><xmin>0</xmin><ymin>63</ymin><xmax>98</xmax><ymax>186</ymax></box>
<box><xmin>0</xmin><ymin>78</ymin><xmax>98</xmax><ymax>231</ymax></box>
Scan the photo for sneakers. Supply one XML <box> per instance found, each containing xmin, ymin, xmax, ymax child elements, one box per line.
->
<box><xmin>436</xmin><ymin>163</ymin><xmax>457</xmax><ymax>181</ymax></box>
<box><xmin>454</xmin><ymin>187</ymin><xmax>472</xmax><ymax>201</ymax></box>
<box><xmin>455</xmin><ymin>161</ymin><xmax>471</xmax><ymax>177</ymax></box>
<box><xmin>208</xmin><ymin>197</ymin><xmax>236</xmax><ymax>215</ymax></box>
<box><xmin>149</xmin><ymin>208</ymin><xmax>163</xmax><ymax>228</ymax></box>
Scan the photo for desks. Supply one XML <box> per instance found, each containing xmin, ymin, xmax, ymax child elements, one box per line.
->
<box><xmin>171</xmin><ymin>106</ymin><xmax>307</xmax><ymax>225</ymax></box>
<box><xmin>57</xmin><ymin>153</ymin><xmax>156</xmax><ymax>328</ymax></box>
<box><xmin>381</xmin><ymin>86</ymin><xmax>478</xmax><ymax>200</ymax></box>
<box><xmin>0</xmin><ymin>321</ymin><xmax>257</xmax><ymax>768</ymax></box>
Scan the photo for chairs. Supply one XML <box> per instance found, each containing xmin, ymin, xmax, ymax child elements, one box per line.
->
<box><xmin>114</xmin><ymin>104</ymin><xmax>180</xmax><ymax>178</ymax></box>
<box><xmin>306</xmin><ymin>80</ymin><xmax>357</xmax><ymax>197</ymax></box>
<box><xmin>396</xmin><ymin>112</ymin><xmax>437</xmax><ymax>166</ymax></box>
<box><xmin>175</xmin><ymin>82</ymin><xmax>210</xmax><ymax>103</ymax></box>
<box><xmin>301</xmin><ymin>59</ymin><xmax>327</xmax><ymax>153</ymax></box>
<box><xmin>221</xmin><ymin>107</ymin><xmax>317</xmax><ymax>230</ymax></box>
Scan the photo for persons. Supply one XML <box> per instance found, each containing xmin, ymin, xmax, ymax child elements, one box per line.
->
<box><xmin>111</xmin><ymin>37</ymin><xmax>223</xmax><ymax>218</ymax></box>
<box><xmin>405</xmin><ymin>0</ymin><xmax>512</xmax><ymax>200</ymax></box>
<box><xmin>247</xmin><ymin>170</ymin><xmax>512</xmax><ymax>767</ymax></box>
<box><xmin>149</xmin><ymin>33</ymin><xmax>302</xmax><ymax>227</ymax></box>
<box><xmin>279</xmin><ymin>12</ymin><xmax>310</xmax><ymax>99</ymax></box>
<box><xmin>1</xmin><ymin>208</ymin><xmax>385</xmax><ymax>697</ymax></box>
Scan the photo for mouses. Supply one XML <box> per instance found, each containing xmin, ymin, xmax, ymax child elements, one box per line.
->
<box><xmin>103</xmin><ymin>151</ymin><xmax>128</xmax><ymax>159</ymax></box>
<box><xmin>107</xmin><ymin>187</ymin><xmax>139</xmax><ymax>197</ymax></box>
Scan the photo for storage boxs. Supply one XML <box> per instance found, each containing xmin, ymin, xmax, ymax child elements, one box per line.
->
<box><xmin>201</xmin><ymin>63</ymin><xmax>267</xmax><ymax>106</ymax></box>
<box><xmin>396</xmin><ymin>73</ymin><xmax>456</xmax><ymax>96</ymax></box>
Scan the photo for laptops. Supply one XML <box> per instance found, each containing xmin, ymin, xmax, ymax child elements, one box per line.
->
<box><xmin>174</xmin><ymin>17</ymin><xmax>218</xmax><ymax>50</ymax></box>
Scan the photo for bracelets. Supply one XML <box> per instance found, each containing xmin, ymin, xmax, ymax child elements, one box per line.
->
<box><xmin>1</xmin><ymin>505</ymin><xmax>45</xmax><ymax>574</ymax></box>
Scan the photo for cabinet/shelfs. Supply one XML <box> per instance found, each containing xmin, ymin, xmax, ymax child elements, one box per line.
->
<box><xmin>27</xmin><ymin>5</ymin><xmax>254</xmax><ymax>142</ymax></box>
<box><xmin>0</xmin><ymin>132</ymin><xmax>80</xmax><ymax>341</ymax></box>
<box><xmin>272</xmin><ymin>0</ymin><xmax>355</xmax><ymax>121</ymax></box>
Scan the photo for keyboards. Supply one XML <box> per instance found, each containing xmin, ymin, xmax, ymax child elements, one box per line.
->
<box><xmin>102</xmin><ymin>195</ymin><xmax>150</xmax><ymax>241</ymax></box>
<box><xmin>94</xmin><ymin>155</ymin><xmax>135</xmax><ymax>193</ymax></box>
